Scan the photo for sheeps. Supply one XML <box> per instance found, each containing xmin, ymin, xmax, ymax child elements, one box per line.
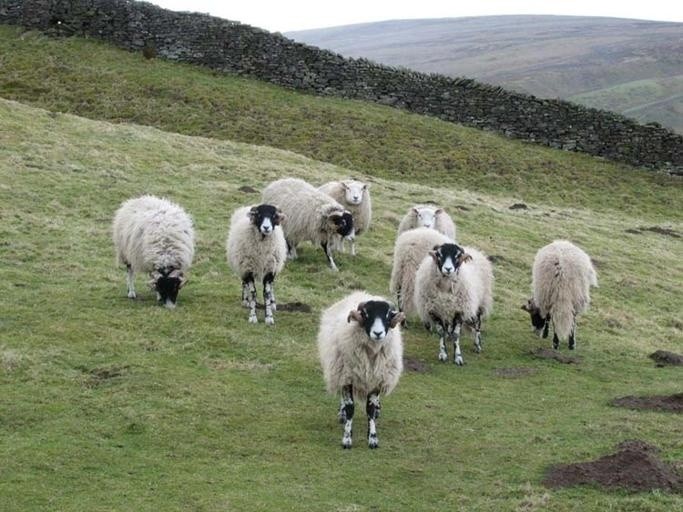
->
<box><xmin>112</xmin><ymin>194</ymin><xmax>196</xmax><ymax>309</ymax></box>
<box><xmin>520</xmin><ymin>239</ymin><xmax>600</xmax><ymax>352</ymax></box>
<box><xmin>388</xmin><ymin>203</ymin><xmax>496</xmax><ymax>367</ymax></box>
<box><xmin>318</xmin><ymin>288</ymin><xmax>404</xmax><ymax>450</ymax></box>
<box><xmin>225</xmin><ymin>203</ymin><xmax>288</xmax><ymax>326</ymax></box>
<box><xmin>260</xmin><ymin>177</ymin><xmax>372</xmax><ymax>273</ymax></box>
